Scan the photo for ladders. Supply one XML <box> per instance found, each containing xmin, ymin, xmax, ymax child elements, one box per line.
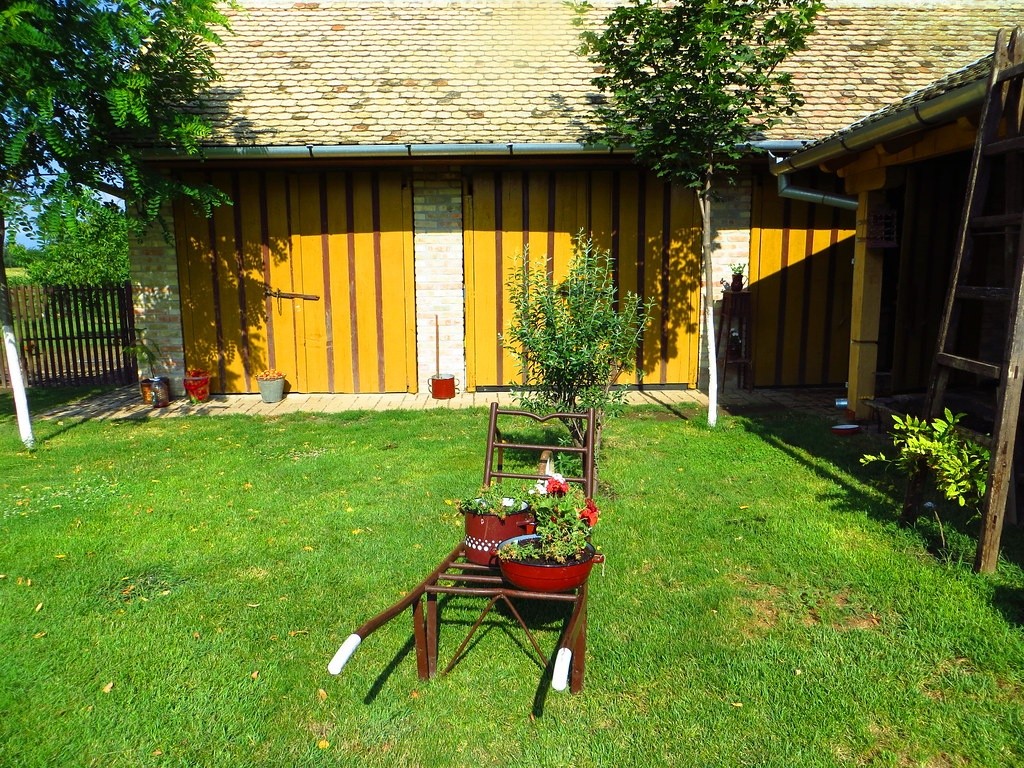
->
<box><xmin>907</xmin><ymin>26</ymin><xmax>1023</xmax><ymax>572</ymax></box>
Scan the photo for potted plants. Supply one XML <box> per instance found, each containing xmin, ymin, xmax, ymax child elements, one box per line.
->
<box><xmin>729</xmin><ymin>265</ymin><xmax>746</xmax><ymax>291</ymax></box>
<box><xmin>121</xmin><ymin>327</ymin><xmax>176</xmax><ymax>406</ymax></box>
<box><xmin>454</xmin><ymin>480</ymin><xmax>542</xmax><ymax>562</ymax></box>
<box><xmin>495</xmin><ymin>525</ymin><xmax>601</xmax><ymax>591</ymax></box>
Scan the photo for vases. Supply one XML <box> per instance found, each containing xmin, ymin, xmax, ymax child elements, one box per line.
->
<box><xmin>730</xmin><ymin>340</ymin><xmax>740</xmax><ymax>360</ymax></box>
<box><xmin>257</xmin><ymin>376</ymin><xmax>285</xmax><ymax>403</ymax></box>
<box><xmin>530</xmin><ymin>516</ymin><xmax>594</xmax><ymax>544</ymax></box>
<box><xmin>184</xmin><ymin>378</ymin><xmax>210</xmax><ymax>403</ymax></box>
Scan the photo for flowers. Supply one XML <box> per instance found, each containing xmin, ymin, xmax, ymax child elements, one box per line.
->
<box><xmin>527</xmin><ymin>472</ymin><xmax>598</xmax><ymax>526</ymax></box>
<box><xmin>255</xmin><ymin>368</ymin><xmax>286</xmax><ymax>379</ymax></box>
<box><xmin>730</xmin><ymin>327</ymin><xmax>741</xmax><ymax>343</ymax></box>
<box><xmin>184</xmin><ymin>368</ymin><xmax>211</xmax><ymax>378</ymax></box>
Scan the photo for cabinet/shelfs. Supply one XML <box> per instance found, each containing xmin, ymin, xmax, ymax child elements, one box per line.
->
<box><xmin>715</xmin><ymin>290</ymin><xmax>752</xmax><ymax>396</ymax></box>
